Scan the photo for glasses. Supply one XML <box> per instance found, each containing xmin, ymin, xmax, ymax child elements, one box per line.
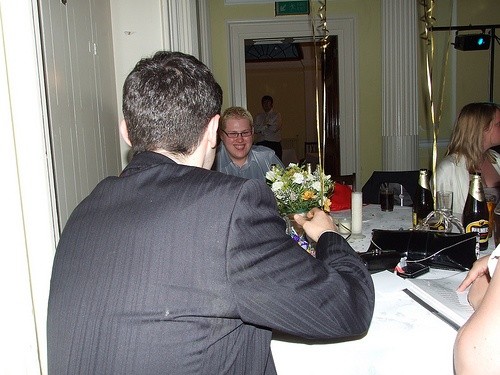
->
<box><xmin>222</xmin><ymin>127</ymin><xmax>252</xmax><ymax>138</ymax></box>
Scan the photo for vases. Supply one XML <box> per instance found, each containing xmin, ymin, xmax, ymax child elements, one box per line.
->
<box><xmin>287</xmin><ymin>213</ymin><xmax>314</xmax><ymax>238</ymax></box>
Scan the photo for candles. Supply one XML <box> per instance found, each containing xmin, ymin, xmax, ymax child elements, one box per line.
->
<box><xmin>351</xmin><ymin>191</ymin><xmax>362</xmax><ymax>234</ymax></box>
<box><xmin>339</xmin><ymin>217</ymin><xmax>350</xmax><ymax>235</ymax></box>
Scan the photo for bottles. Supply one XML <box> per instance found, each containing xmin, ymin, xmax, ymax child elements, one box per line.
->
<box><xmin>462</xmin><ymin>171</ymin><xmax>490</xmax><ymax>250</ymax></box>
<box><xmin>265</xmin><ymin>163</ymin><xmax>279</xmax><ymax>189</ymax></box>
<box><xmin>411</xmin><ymin>168</ymin><xmax>435</xmax><ymax>229</ymax></box>
<box><xmin>306</xmin><ymin>162</ymin><xmax>313</xmax><ymax>175</ymax></box>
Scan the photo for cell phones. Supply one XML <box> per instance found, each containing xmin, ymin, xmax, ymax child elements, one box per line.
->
<box><xmin>397</xmin><ymin>262</ymin><xmax>430</xmax><ymax>279</ymax></box>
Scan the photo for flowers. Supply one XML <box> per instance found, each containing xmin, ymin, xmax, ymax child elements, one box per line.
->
<box><xmin>266</xmin><ymin>162</ymin><xmax>334</xmax><ymax>258</ymax></box>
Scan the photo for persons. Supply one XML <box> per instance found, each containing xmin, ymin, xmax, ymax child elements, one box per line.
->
<box><xmin>211</xmin><ymin>106</ymin><xmax>285</xmax><ymax>186</ymax></box>
<box><xmin>430</xmin><ymin>101</ymin><xmax>500</xmax><ymax>234</ymax></box>
<box><xmin>46</xmin><ymin>50</ymin><xmax>376</xmax><ymax>375</ymax></box>
<box><xmin>454</xmin><ymin>244</ymin><xmax>500</xmax><ymax>375</ymax></box>
<box><xmin>254</xmin><ymin>95</ymin><xmax>283</xmax><ymax>161</ymax></box>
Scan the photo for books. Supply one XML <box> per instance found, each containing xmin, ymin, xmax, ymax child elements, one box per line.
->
<box><xmin>401</xmin><ymin>271</ymin><xmax>475</xmax><ymax>331</ymax></box>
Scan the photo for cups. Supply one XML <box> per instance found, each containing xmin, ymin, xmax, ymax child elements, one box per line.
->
<box><xmin>485</xmin><ymin>194</ymin><xmax>500</xmax><ymax>248</ymax></box>
<box><xmin>436</xmin><ymin>191</ymin><xmax>455</xmax><ymax>232</ymax></box>
<box><xmin>379</xmin><ymin>184</ymin><xmax>394</xmax><ymax>212</ymax></box>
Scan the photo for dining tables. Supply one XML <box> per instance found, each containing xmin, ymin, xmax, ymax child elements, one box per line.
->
<box><xmin>268</xmin><ymin>203</ymin><xmax>496</xmax><ymax>375</ymax></box>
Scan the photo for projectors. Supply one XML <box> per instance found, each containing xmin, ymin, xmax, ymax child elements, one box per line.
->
<box><xmin>452</xmin><ymin>33</ymin><xmax>490</xmax><ymax>52</ymax></box>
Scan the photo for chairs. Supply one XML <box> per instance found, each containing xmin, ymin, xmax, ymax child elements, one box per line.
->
<box><xmin>330</xmin><ymin>168</ymin><xmax>436</xmax><ymax>224</ymax></box>
